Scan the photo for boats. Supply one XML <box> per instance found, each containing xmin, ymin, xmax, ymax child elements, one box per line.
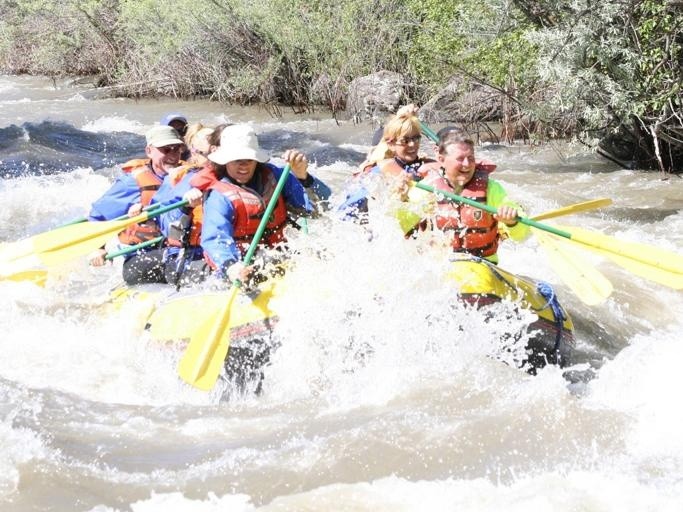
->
<box><xmin>0</xmin><ymin>219</ymin><xmax>580</xmax><ymax>419</ymax></box>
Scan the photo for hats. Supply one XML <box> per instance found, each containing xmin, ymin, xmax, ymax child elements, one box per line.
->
<box><xmin>146</xmin><ymin>125</ymin><xmax>185</xmax><ymax>147</ymax></box>
<box><xmin>192</xmin><ymin>127</ymin><xmax>213</xmax><ymax>152</ymax></box>
<box><xmin>160</xmin><ymin>113</ymin><xmax>186</xmax><ymax>126</ymax></box>
<box><xmin>207</xmin><ymin>125</ymin><xmax>269</xmax><ymax>165</ymax></box>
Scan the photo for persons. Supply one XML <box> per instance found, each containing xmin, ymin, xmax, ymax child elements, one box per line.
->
<box><xmin>336</xmin><ymin>116</ymin><xmax>437</xmax><ymax>244</ymax></box>
<box><xmin>354</xmin><ymin>103</ymin><xmax>421</xmax><ymax>177</ymax></box>
<box><xmin>392</xmin><ymin>129</ymin><xmax>532</xmax><ymax>265</ymax></box>
<box><xmin>85</xmin><ymin>113</ymin><xmax>330</xmax><ymax>293</ymax></box>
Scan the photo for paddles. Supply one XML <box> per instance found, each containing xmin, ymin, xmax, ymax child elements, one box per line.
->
<box><xmin>2</xmin><ymin>198</ymin><xmax>190</xmax><ymax>289</ymax></box>
<box><xmin>178</xmin><ymin>161</ymin><xmax>293</xmax><ymax>390</ymax></box>
<box><xmin>407</xmin><ymin>180</ymin><xmax>683</xmax><ymax>279</ymax></box>
<box><xmin>529</xmin><ymin>198</ymin><xmax>611</xmax><ymax>221</ymax></box>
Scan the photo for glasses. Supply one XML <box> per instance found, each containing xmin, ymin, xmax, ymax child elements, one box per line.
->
<box><xmin>401</xmin><ymin>135</ymin><xmax>421</xmax><ymax>145</ymax></box>
<box><xmin>159</xmin><ymin>145</ymin><xmax>181</xmax><ymax>154</ymax></box>
